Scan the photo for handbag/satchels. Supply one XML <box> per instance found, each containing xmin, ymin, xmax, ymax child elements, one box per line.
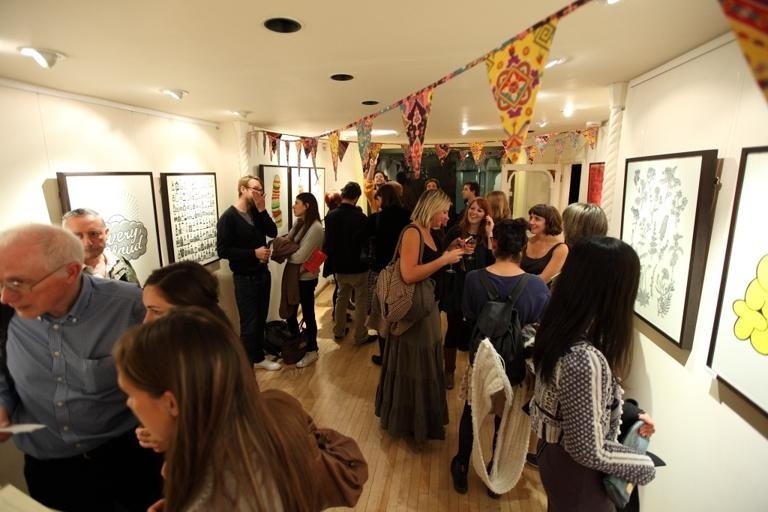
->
<box><xmin>261</xmin><ymin>318</ymin><xmax>309</xmax><ymax>365</ymax></box>
<box><xmin>303</xmin><ymin>249</ymin><xmax>327</xmax><ymax>272</ymax></box>
<box><xmin>390</xmin><ymin>260</ymin><xmax>436</xmax><ymax>335</ymax></box>
<box><xmin>603</xmin><ymin>420</ymin><xmax>650</xmax><ymax>505</ymax></box>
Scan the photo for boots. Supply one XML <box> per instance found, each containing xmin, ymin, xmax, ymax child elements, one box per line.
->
<box><xmin>372</xmin><ymin>333</ymin><xmax>385</xmax><ymax>367</ymax></box>
<box><xmin>445</xmin><ymin>358</ymin><xmax>456</xmax><ymax>389</ymax></box>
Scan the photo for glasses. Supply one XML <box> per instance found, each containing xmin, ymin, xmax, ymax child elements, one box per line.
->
<box><xmin>244</xmin><ymin>186</ymin><xmax>263</xmax><ymax>193</ymax></box>
<box><xmin>0</xmin><ymin>266</ymin><xmax>66</xmax><ymax>294</ymax></box>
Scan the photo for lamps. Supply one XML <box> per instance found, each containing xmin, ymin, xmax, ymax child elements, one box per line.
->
<box><xmin>21</xmin><ymin>47</ymin><xmax>65</xmax><ymax>68</ymax></box>
<box><xmin>159</xmin><ymin>88</ymin><xmax>188</xmax><ymax>99</ymax></box>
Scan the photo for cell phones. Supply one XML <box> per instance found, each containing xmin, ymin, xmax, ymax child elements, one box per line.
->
<box><xmin>463</xmin><ymin>235</ymin><xmax>474</xmax><ymax>243</ymax></box>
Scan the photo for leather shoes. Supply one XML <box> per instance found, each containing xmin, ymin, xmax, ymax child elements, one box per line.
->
<box><xmin>361</xmin><ymin>334</ymin><xmax>377</xmax><ymax>344</ymax></box>
<box><xmin>449</xmin><ymin>453</ymin><xmax>469</xmax><ymax>493</ymax></box>
<box><xmin>335</xmin><ymin>328</ymin><xmax>350</xmax><ymax>340</ymax></box>
<box><xmin>333</xmin><ymin>316</ymin><xmax>352</xmax><ymax>322</ymax></box>
<box><xmin>347</xmin><ymin>302</ymin><xmax>356</xmax><ymax>310</ymax></box>
<box><xmin>332</xmin><ymin>311</ymin><xmax>351</xmax><ymax>317</ymax></box>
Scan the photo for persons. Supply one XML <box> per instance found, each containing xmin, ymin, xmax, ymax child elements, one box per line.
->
<box><xmin>214</xmin><ymin>174</ymin><xmax>285</xmax><ymax>371</ymax></box>
<box><xmin>135</xmin><ymin>259</ymin><xmax>237</xmax><ymax>453</ymax></box>
<box><xmin>323</xmin><ymin>169</ymin><xmax>610</xmax><ymax>373</ymax></box>
<box><xmin>384</xmin><ymin>188</ymin><xmax>467</xmax><ymax>443</ymax></box>
<box><xmin>0</xmin><ymin>222</ymin><xmax>174</xmax><ymax>511</ymax></box>
<box><xmin>109</xmin><ymin>307</ymin><xmax>324</xmax><ymax>512</ymax></box>
<box><xmin>273</xmin><ymin>191</ymin><xmax>324</xmax><ymax>368</ymax></box>
<box><xmin>61</xmin><ymin>208</ymin><xmax>141</xmax><ymax>287</ymax></box>
<box><xmin>450</xmin><ymin>217</ymin><xmax>550</xmax><ymax>498</ymax></box>
<box><xmin>523</xmin><ymin>235</ymin><xmax>657</xmax><ymax>511</ymax></box>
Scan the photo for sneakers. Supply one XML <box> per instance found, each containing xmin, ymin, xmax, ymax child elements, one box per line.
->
<box><xmin>253</xmin><ymin>359</ymin><xmax>280</xmax><ymax>371</ymax></box>
<box><xmin>524</xmin><ymin>450</ymin><xmax>539</xmax><ymax>468</ymax></box>
<box><xmin>296</xmin><ymin>351</ymin><xmax>318</xmax><ymax>367</ymax></box>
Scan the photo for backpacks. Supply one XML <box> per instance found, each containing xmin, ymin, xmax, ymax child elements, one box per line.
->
<box><xmin>470</xmin><ymin>269</ymin><xmax>528</xmax><ymax>386</ymax></box>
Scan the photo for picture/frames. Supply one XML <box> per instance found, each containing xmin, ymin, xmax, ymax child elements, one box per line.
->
<box><xmin>587</xmin><ymin>160</ymin><xmax>607</xmax><ymax>207</ymax></box>
<box><xmin>619</xmin><ymin>145</ymin><xmax>719</xmax><ymax>350</ymax></box>
<box><xmin>259</xmin><ymin>165</ymin><xmax>326</xmax><ymax>246</ymax></box>
<box><xmin>59</xmin><ymin>172</ymin><xmax>220</xmax><ymax>286</ymax></box>
<box><xmin>706</xmin><ymin>147</ymin><xmax>768</xmax><ymax>420</ymax></box>
